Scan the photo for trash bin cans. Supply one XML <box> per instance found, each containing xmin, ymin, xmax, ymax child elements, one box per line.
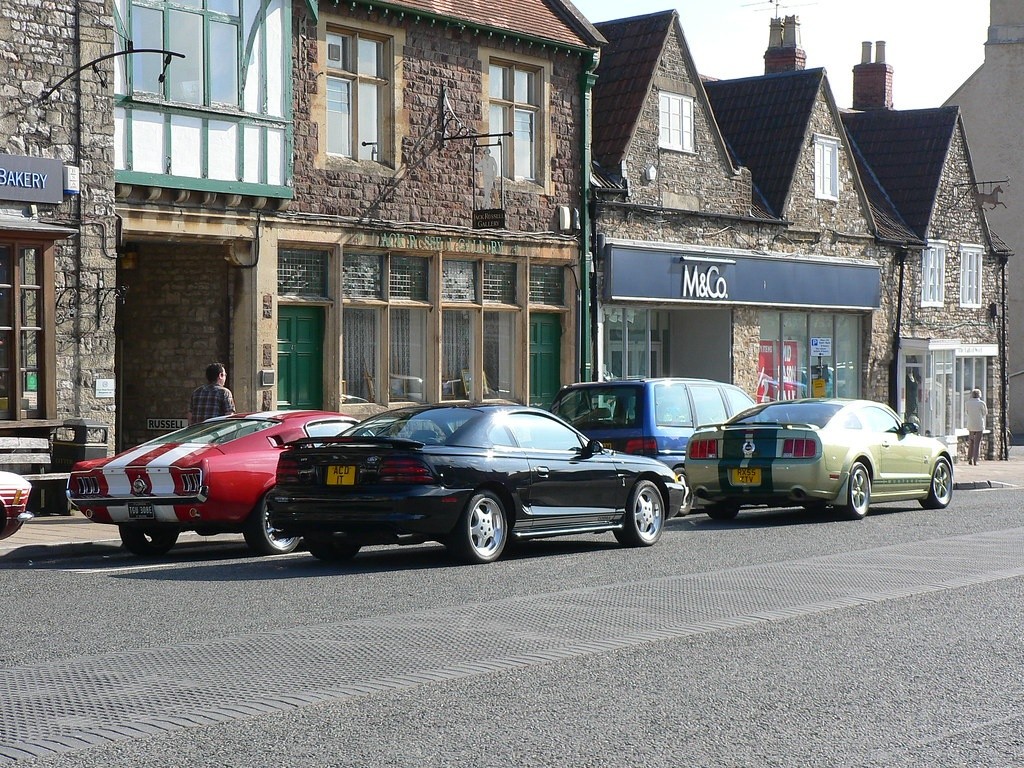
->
<box><xmin>40</xmin><ymin>418</ymin><xmax>109</xmax><ymax>515</ymax></box>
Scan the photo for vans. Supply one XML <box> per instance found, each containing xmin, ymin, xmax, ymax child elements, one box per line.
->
<box><xmin>548</xmin><ymin>378</ymin><xmax>759</xmax><ymax>516</ymax></box>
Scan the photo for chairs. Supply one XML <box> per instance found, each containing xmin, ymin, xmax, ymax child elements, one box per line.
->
<box><xmin>591</xmin><ymin>407</ymin><xmax>620</xmax><ymax>424</ymax></box>
<box><xmin>409</xmin><ymin>430</ymin><xmax>440</xmax><ymax>442</ymax></box>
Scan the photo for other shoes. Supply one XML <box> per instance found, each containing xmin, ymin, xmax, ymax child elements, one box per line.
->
<box><xmin>973</xmin><ymin>459</ymin><xmax>976</xmax><ymax>465</ymax></box>
<box><xmin>969</xmin><ymin>460</ymin><xmax>972</xmax><ymax>464</ymax></box>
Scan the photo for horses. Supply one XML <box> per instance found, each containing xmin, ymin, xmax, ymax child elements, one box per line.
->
<box><xmin>968</xmin><ymin>185</ymin><xmax>1007</xmax><ymax>213</ymax></box>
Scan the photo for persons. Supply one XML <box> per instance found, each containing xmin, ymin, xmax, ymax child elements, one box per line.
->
<box><xmin>187</xmin><ymin>363</ymin><xmax>235</xmax><ymax>425</ymax></box>
<box><xmin>965</xmin><ymin>389</ymin><xmax>987</xmax><ymax>465</ymax></box>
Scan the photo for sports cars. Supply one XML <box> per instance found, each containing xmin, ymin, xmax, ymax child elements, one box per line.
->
<box><xmin>685</xmin><ymin>397</ymin><xmax>955</xmax><ymax>520</ymax></box>
<box><xmin>66</xmin><ymin>410</ymin><xmax>362</xmax><ymax>554</ymax></box>
<box><xmin>275</xmin><ymin>402</ymin><xmax>685</xmax><ymax>563</ymax></box>
